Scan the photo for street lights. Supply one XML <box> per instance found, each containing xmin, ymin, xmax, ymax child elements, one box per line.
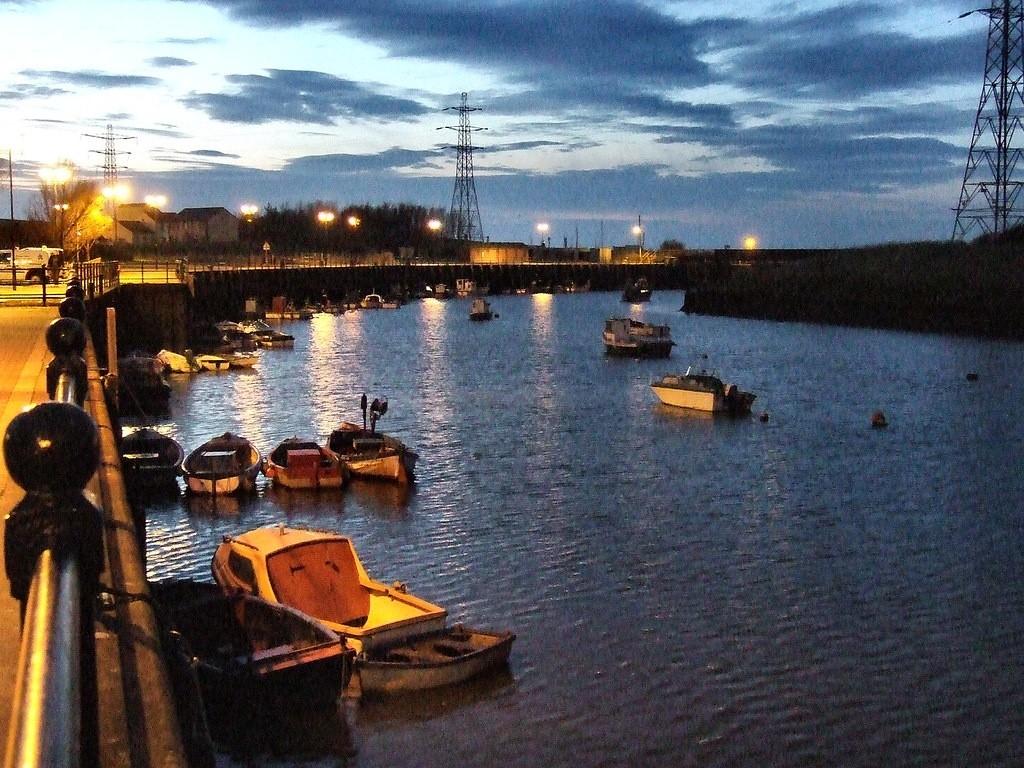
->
<box><xmin>634</xmin><ymin>227</ymin><xmax>643</xmax><ymax>260</ymax></box>
<box><xmin>318</xmin><ymin>210</ymin><xmax>334</xmax><ymax>242</ymax></box>
<box><xmin>538</xmin><ymin>223</ymin><xmax>548</xmax><ymax>242</ymax></box>
<box><xmin>241</xmin><ymin>202</ymin><xmax>258</xmax><ymax>267</ymax></box>
<box><xmin>145</xmin><ymin>194</ymin><xmax>168</xmax><ymax>269</ymax></box>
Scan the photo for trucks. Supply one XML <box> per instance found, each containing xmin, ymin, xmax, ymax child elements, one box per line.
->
<box><xmin>0</xmin><ymin>246</ymin><xmax>64</xmax><ymax>284</ymax></box>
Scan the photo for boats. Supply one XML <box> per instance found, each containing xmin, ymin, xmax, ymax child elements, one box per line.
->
<box><xmin>180</xmin><ymin>432</ymin><xmax>262</xmax><ymax>498</ymax></box>
<box><xmin>650</xmin><ymin>366</ymin><xmax>757</xmax><ymax>414</ymax></box>
<box><xmin>145</xmin><ymin>576</ymin><xmax>359</xmax><ymax>731</ymax></box>
<box><xmin>155</xmin><ymin>292</ymin><xmax>401</xmax><ymax>375</ymax></box>
<box><xmin>350</xmin><ymin>622</ymin><xmax>518</xmax><ymax>694</ymax></box>
<box><xmin>208</xmin><ymin>522</ymin><xmax>449</xmax><ymax>691</ymax></box>
<box><xmin>262</xmin><ymin>437</ymin><xmax>349</xmax><ymax>491</ymax></box>
<box><xmin>603</xmin><ymin>319</ymin><xmax>676</xmax><ymax>358</ymax></box>
<box><xmin>470</xmin><ymin>298</ymin><xmax>495</xmax><ymax>321</ymax></box>
<box><xmin>325</xmin><ymin>395</ymin><xmax>419</xmax><ymax>485</ymax></box>
<box><xmin>117</xmin><ymin>427</ymin><xmax>186</xmax><ymax>500</ymax></box>
